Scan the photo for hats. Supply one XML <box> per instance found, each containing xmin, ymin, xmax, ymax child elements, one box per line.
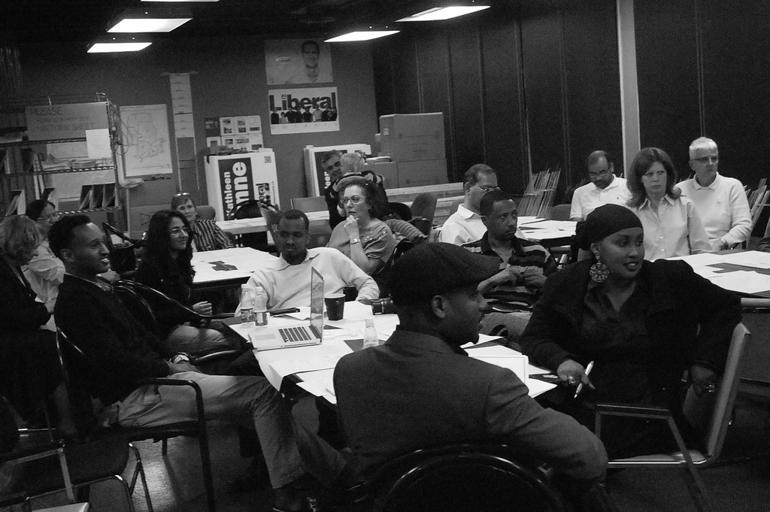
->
<box><xmin>387</xmin><ymin>241</ymin><xmax>501</xmax><ymax>305</ymax></box>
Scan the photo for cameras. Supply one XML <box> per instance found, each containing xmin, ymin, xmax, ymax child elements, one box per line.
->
<box><xmin>372</xmin><ymin>297</ymin><xmax>396</xmax><ymax>314</ymax></box>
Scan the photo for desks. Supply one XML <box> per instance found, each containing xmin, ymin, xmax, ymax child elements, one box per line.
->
<box><xmin>490</xmin><ymin>214</ymin><xmax>578</xmax><ymax>240</ymax></box>
<box><xmin>203</xmin><ymin>211</ymin><xmax>336</xmax><ymax>237</ymax></box>
<box><xmin>173</xmin><ymin>247</ymin><xmax>290</xmax><ymax>289</ymax></box>
<box><xmin>326</xmin><ymin>182</ymin><xmax>472</xmax><ymax>209</ymax></box>
<box><xmin>656</xmin><ymin>242</ymin><xmax>768</xmax><ymax>403</ymax></box>
<box><xmin>223</xmin><ymin>305</ymin><xmax>556</xmax><ymax>414</ymax></box>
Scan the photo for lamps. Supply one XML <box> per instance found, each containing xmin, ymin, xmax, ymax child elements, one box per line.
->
<box><xmin>392</xmin><ymin>1</ymin><xmax>497</xmax><ymax>26</ymax></box>
<box><xmin>316</xmin><ymin>9</ymin><xmax>401</xmax><ymax>45</ymax></box>
<box><xmin>60</xmin><ymin>17</ymin><xmax>159</xmax><ymax>60</ymax></box>
<box><xmin>102</xmin><ymin>1</ymin><xmax>192</xmax><ymax>34</ymax></box>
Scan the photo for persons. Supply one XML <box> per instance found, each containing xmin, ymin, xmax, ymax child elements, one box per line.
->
<box><xmin>270</xmin><ymin>103</ymin><xmax>337</xmax><ymax>123</ymax></box>
<box><xmin>0</xmin><ymin>134</ymin><xmax>769</xmax><ymax>512</ymax></box>
<box><xmin>286</xmin><ymin>41</ymin><xmax>333</xmax><ymax>84</ymax></box>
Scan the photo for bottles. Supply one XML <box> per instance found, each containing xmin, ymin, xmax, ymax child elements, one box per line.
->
<box><xmin>362</xmin><ymin>316</ymin><xmax>380</xmax><ymax>349</ymax></box>
<box><xmin>252</xmin><ymin>286</ymin><xmax>268</xmax><ymax>333</ymax></box>
<box><xmin>239</xmin><ymin>283</ymin><xmax>253</xmax><ymax>329</ymax></box>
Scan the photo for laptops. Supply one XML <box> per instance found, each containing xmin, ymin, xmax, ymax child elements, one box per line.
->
<box><xmin>247</xmin><ymin>264</ymin><xmax>326</xmax><ymax>351</ymax></box>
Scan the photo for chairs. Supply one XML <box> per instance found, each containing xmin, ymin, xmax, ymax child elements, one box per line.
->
<box><xmin>2</xmin><ymin>441</ymin><xmax>91</xmax><ymax>512</ymax></box>
<box><xmin>2</xmin><ymin>391</ymin><xmax>156</xmax><ymax>512</ymax></box>
<box><xmin>293</xmin><ymin>192</ymin><xmax>334</xmax><ymax>247</ymax></box>
<box><xmin>405</xmin><ymin>194</ymin><xmax>442</xmax><ymax>238</ymax></box>
<box><xmin>362</xmin><ymin>453</ymin><xmax>565</xmax><ymax>512</ymax></box>
<box><xmin>578</xmin><ymin>321</ymin><xmax>752</xmax><ymax>512</ymax></box>
<box><xmin>256</xmin><ymin>201</ymin><xmax>296</xmax><ymax>246</ymax></box>
<box><xmin>104</xmin><ymin>218</ymin><xmax>146</xmax><ymax>254</ymax></box>
<box><xmin>297</xmin><ymin>439</ymin><xmax>521</xmax><ymax>510</ymax></box>
<box><xmin>53</xmin><ymin>322</ymin><xmax>216</xmax><ymax>512</ymax></box>
<box><xmin>186</xmin><ymin>202</ymin><xmax>217</xmax><ymax>223</ymax></box>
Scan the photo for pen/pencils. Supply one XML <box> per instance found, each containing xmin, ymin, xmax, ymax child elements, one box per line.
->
<box><xmin>574</xmin><ymin>360</ymin><xmax>595</xmax><ymax>399</ymax></box>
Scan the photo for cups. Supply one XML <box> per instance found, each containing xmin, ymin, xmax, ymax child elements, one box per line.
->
<box><xmin>324</xmin><ymin>293</ymin><xmax>347</xmax><ymax>321</ymax></box>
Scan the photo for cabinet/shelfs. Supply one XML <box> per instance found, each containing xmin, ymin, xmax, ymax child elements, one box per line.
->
<box><xmin>380</xmin><ymin>112</ymin><xmax>454</xmax><ymax>183</ymax></box>
<box><xmin>2</xmin><ymin>97</ymin><xmax>133</xmax><ymax>242</ymax></box>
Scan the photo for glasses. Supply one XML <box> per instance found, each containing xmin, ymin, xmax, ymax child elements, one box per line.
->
<box><xmin>588</xmin><ymin>170</ymin><xmax>608</xmax><ymax>179</ymax></box>
<box><xmin>343</xmin><ymin>195</ymin><xmax>360</xmax><ymax>203</ymax></box>
<box><xmin>173</xmin><ymin>192</ymin><xmax>191</xmax><ymax>198</ymax></box>
<box><xmin>481</xmin><ymin>187</ymin><xmax>501</xmax><ymax>192</ymax></box>
<box><xmin>693</xmin><ymin>156</ymin><xmax>716</xmax><ymax>162</ymax></box>
